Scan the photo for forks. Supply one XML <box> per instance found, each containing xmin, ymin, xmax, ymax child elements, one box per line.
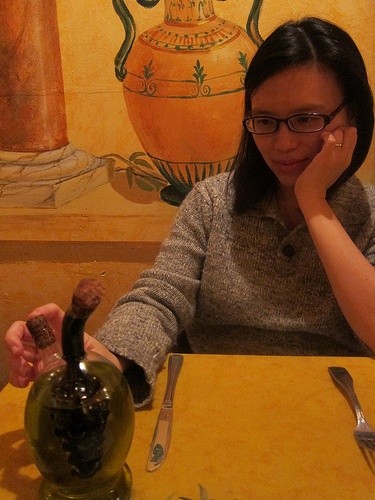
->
<box><xmin>328</xmin><ymin>366</ymin><xmax>375</xmax><ymax>450</ymax></box>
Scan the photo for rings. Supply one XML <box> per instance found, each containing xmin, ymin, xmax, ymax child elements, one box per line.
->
<box><xmin>335</xmin><ymin>143</ymin><xmax>342</xmax><ymax>148</ymax></box>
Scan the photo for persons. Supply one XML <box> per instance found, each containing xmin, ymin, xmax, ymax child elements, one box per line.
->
<box><xmin>5</xmin><ymin>15</ymin><xmax>375</xmax><ymax>409</ymax></box>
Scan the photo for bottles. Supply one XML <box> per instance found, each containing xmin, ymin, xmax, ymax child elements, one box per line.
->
<box><xmin>23</xmin><ymin>277</ymin><xmax>136</xmax><ymax>500</ymax></box>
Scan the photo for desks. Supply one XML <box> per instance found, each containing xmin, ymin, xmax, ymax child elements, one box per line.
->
<box><xmin>0</xmin><ymin>353</ymin><xmax>375</xmax><ymax>500</ymax></box>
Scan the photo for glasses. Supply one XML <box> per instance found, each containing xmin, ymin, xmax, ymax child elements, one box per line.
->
<box><xmin>243</xmin><ymin>102</ymin><xmax>346</xmax><ymax>135</ymax></box>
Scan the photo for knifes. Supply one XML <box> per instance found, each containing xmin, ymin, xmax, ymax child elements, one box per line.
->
<box><xmin>146</xmin><ymin>355</ymin><xmax>183</xmax><ymax>472</ymax></box>
